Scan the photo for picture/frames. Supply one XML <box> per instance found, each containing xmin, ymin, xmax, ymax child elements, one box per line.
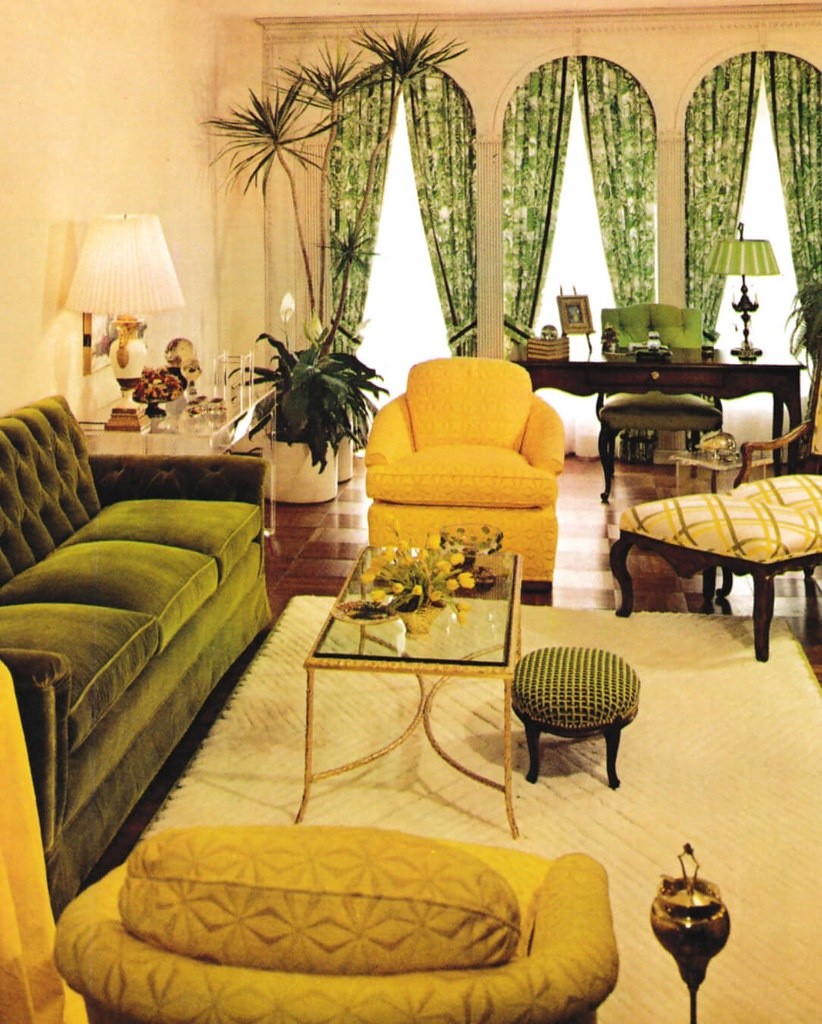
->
<box><xmin>556</xmin><ymin>295</ymin><xmax>595</xmax><ymax>334</ymax></box>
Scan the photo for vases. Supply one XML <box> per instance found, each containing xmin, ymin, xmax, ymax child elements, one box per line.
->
<box><xmin>132</xmin><ymin>391</ymin><xmax>174</xmax><ymax>418</ymax></box>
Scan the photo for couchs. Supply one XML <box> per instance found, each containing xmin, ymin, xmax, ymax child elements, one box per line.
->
<box><xmin>53</xmin><ymin>827</ymin><xmax>618</xmax><ymax>1024</ymax></box>
<box><xmin>365</xmin><ymin>356</ymin><xmax>565</xmax><ymax>592</ymax></box>
<box><xmin>0</xmin><ymin>396</ymin><xmax>273</xmax><ymax>923</ymax></box>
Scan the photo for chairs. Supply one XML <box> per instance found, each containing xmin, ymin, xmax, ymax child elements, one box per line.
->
<box><xmin>596</xmin><ymin>303</ymin><xmax>724</xmax><ymax>501</ymax></box>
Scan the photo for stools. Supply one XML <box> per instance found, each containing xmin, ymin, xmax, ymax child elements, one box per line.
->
<box><xmin>715</xmin><ymin>474</ymin><xmax>822</xmax><ymax>599</ymax></box>
<box><xmin>511</xmin><ymin>646</ymin><xmax>641</xmax><ymax>791</ymax></box>
<box><xmin>609</xmin><ymin>493</ymin><xmax>822</xmax><ymax>663</ymax></box>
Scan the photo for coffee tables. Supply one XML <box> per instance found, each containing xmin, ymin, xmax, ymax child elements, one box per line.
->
<box><xmin>296</xmin><ymin>546</ymin><xmax>523</xmax><ymax>840</ymax></box>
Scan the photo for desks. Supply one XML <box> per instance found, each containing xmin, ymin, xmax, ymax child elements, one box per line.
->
<box><xmin>671</xmin><ymin>454</ymin><xmax>773</xmax><ymax>490</ymax></box>
<box><xmin>506</xmin><ymin>346</ymin><xmax>808</xmax><ymax>476</ymax></box>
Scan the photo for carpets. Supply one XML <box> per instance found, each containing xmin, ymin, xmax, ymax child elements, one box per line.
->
<box><xmin>138</xmin><ymin>595</ymin><xmax>822</xmax><ymax>1024</ymax></box>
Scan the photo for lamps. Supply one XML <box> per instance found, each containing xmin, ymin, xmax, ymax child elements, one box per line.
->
<box><xmin>709</xmin><ymin>222</ymin><xmax>780</xmax><ymax>361</ymax></box>
<box><xmin>64</xmin><ymin>213</ymin><xmax>184</xmax><ymax>433</ymax></box>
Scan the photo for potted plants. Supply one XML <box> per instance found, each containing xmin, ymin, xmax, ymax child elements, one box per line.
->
<box><xmin>228</xmin><ymin>291</ymin><xmax>389</xmax><ymax>505</ymax></box>
<box><xmin>201</xmin><ymin>12</ymin><xmax>468</xmax><ymax>484</ymax></box>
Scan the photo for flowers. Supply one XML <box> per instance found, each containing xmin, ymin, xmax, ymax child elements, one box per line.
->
<box><xmin>136</xmin><ymin>366</ymin><xmax>183</xmax><ymax>400</ymax></box>
<box><xmin>361</xmin><ymin>513</ymin><xmax>475</xmax><ymax>623</ymax></box>
